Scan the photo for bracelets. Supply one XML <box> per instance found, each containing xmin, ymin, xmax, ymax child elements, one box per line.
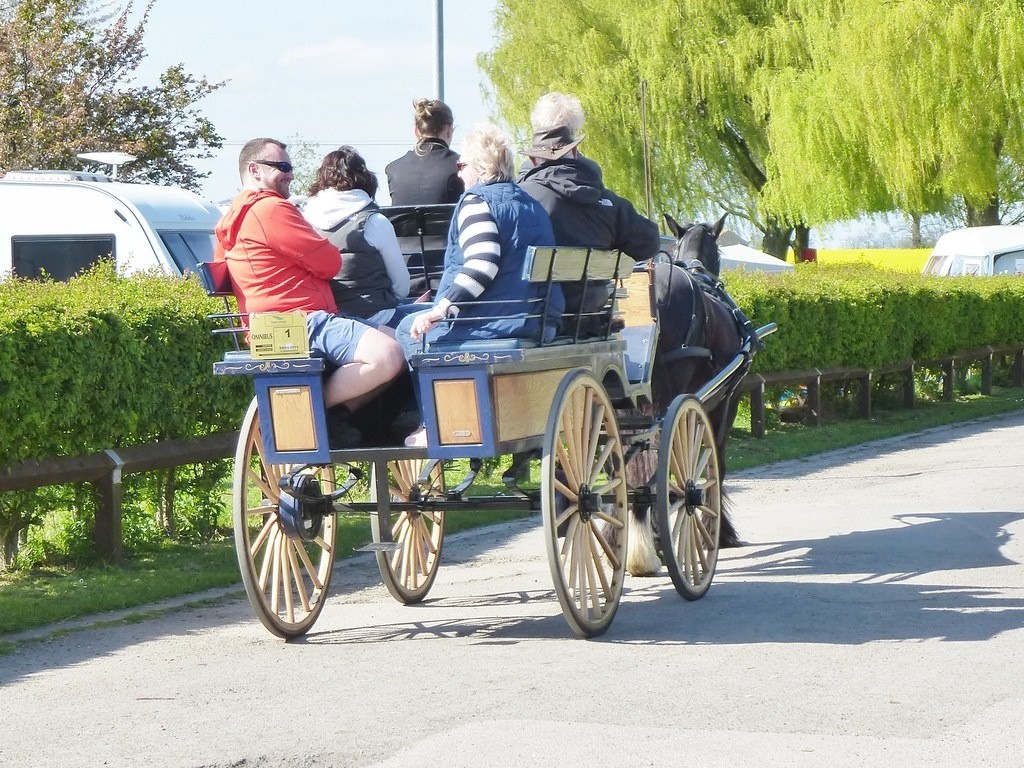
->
<box><xmin>416</xmin><ymin>134</ymin><xmax>446</xmax><ymax>159</ymax></box>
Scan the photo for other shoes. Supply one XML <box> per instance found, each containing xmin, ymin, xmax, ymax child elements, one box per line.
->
<box><xmin>324</xmin><ymin>407</ymin><xmax>363</xmax><ymax>450</ymax></box>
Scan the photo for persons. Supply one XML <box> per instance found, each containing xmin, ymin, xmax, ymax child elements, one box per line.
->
<box><xmin>512</xmin><ymin>127</ymin><xmax>660</xmax><ymax>331</ymax></box>
<box><xmin>516</xmin><ymin>89</ymin><xmax>603</xmax><ymax>185</ymax></box>
<box><xmin>385</xmin><ymin>97</ymin><xmax>464</xmax><ymax>206</ymax></box>
<box><xmin>396</xmin><ymin>124</ymin><xmax>565</xmax><ymax>446</ymax></box>
<box><xmin>296</xmin><ymin>146</ymin><xmax>437</xmax><ymax>444</ymax></box>
<box><xmin>214</xmin><ymin>142</ymin><xmax>406</xmax><ymax>448</ymax></box>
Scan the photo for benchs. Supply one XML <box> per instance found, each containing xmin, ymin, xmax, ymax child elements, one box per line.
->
<box><xmin>418</xmin><ymin>248</ymin><xmax>637</xmax><ymax>444</ymax></box>
<box><xmin>380</xmin><ymin>203</ymin><xmax>453</xmax><ymax>296</ymax></box>
<box><xmin>198</xmin><ymin>253</ymin><xmax>445</xmax><ymax>457</ymax></box>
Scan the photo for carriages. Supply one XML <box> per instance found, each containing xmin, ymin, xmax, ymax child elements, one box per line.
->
<box><xmin>205</xmin><ymin>208</ymin><xmax>781</xmax><ymax>645</ymax></box>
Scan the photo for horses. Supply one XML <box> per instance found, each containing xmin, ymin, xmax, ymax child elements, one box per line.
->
<box><xmin>599</xmin><ymin>210</ymin><xmax>743</xmax><ymax>577</ymax></box>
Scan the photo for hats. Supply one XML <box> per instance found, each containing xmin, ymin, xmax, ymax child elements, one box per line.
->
<box><xmin>518</xmin><ymin>125</ymin><xmax>586</xmax><ymax>160</ymax></box>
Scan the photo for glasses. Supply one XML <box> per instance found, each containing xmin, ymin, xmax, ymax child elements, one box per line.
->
<box><xmin>247</xmin><ymin>159</ymin><xmax>294</xmax><ymax>173</ymax></box>
<box><xmin>456</xmin><ymin>163</ymin><xmax>470</xmax><ymax>171</ymax></box>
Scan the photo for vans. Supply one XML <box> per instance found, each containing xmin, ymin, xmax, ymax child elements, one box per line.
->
<box><xmin>0</xmin><ymin>150</ymin><xmax>232</xmax><ymax>303</ymax></box>
<box><xmin>921</xmin><ymin>224</ymin><xmax>1024</xmax><ymax>282</ymax></box>
